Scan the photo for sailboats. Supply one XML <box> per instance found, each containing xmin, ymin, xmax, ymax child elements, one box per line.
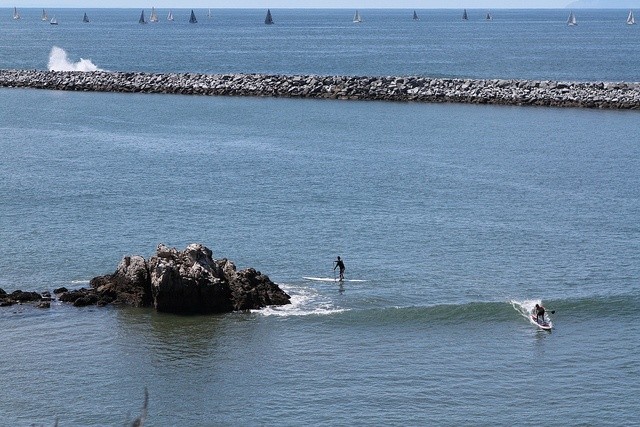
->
<box><xmin>413</xmin><ymin>10</ymin><xmax>420</xmax><ymax>20</ymax></box>
<box><xmin>149</xmin><ymin>6</ymin><xmax>159</xmax><ymax>21</ymax></box>
<box><xmin>462</xmin><ymin>9</ymin><xmax>468</xmax><ymax>18</ymax></box>
<box><xmin>41</xmin><ymin>8</ymin><xmax>48</xmax><ymax>21</ymax></box>
<box><xmin>12</xmin><ymin>6</ymin><xmax>20</xmax><ymax>19</ymax></box>
<box><xmin>189</xmin><ymin>9</ymin><xmax>198</xmax><ymax>23</ymax></box>
<box><xmin>487</xmin><ymin>12</ymin><xmax>491</xmax><ymax>18</ymax></box>
<box><xmin>167</xmin><ymin>11</ymin><xmax>174</xmax><ymax>20</ymax></box>
<box><xmin>264</xmin><ymin>8</ymin><xmax>274</xmax><ymax>24</ymax></box>
<box><xmin>208</xmin><ymin>7</ymin><xmax>210</xmax><ymax>17</ymax></box>
<box><xmin>139</xmin><ymin>9</ymin><xmax>148</xmax><ymax>24</ymax></box>
<box><xmin>626</xmin><ymin>10</ymin><xmax>635</xmax><ymax>25</ymax></box>
<box><xmin>82</xmin><ymin>13</ymin><xmax>89</xmax><ymax>22</ymax></box>
<box><xmin>566</xmin><ymin>10</ymin><xmax>578</xmax><ymax>26</ymax></box>
<box><xmin>352</xmin><ymin>9</ymin><xmax>362</xmax><ymax>23</ymax></box>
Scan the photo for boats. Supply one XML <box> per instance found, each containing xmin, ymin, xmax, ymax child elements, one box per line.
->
<box><xmin>302</xmin><ymin>276</ymin><xmax>369</xmax><ymax>282</ymax></box>
<box><xmin>50</xmin><ymin>17</ymin><xmax>58</xmax><ymax>24</ymax></box>
<box><xmin>530</xmin><ymin>314</ymin><xmax>553</xmax><ymax>330</ymax></box>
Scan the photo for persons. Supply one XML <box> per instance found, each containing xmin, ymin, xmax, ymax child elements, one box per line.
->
<box><xmin>333</xmin><ymin>256</ymin><xmax>345</xmax><ymax>281</ymax></box>
<box><xmin>536</xmin><ymin>303</ymin><xmax>544</xmax><ymax>325</ymax></box>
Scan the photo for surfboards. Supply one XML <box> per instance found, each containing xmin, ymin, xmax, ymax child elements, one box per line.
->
<box><xmin>531</xmin><ymin>314</ymin><xmax>551</xmax><ymax>329</ymax></box>
<box><xmin>303</xmin><ymin>276</ymin><xmax>367</xmax><ymax>281</ymax></box>
